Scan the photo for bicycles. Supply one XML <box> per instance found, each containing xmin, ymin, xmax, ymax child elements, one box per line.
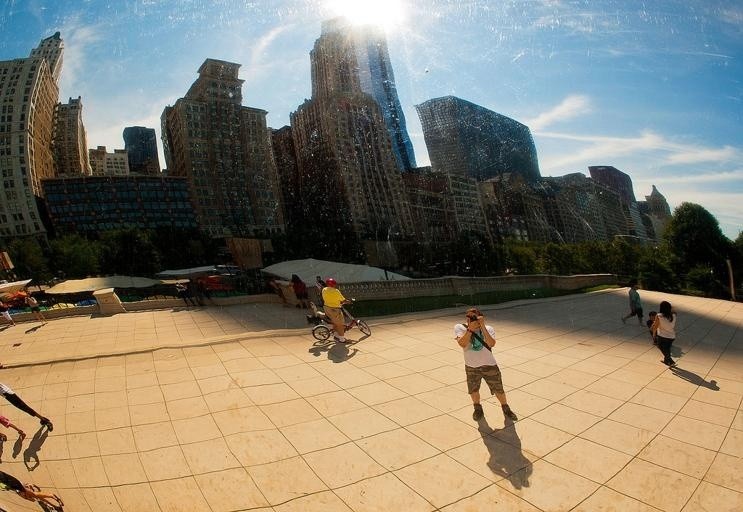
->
<box><xmin>311</xmin><ymin>296</ymin><xmax>373</xmax><ymax>341</ymax></box>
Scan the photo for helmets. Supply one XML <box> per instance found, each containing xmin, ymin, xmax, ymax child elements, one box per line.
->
<box><xmin>325</xmin><ymin>278</ymin><xmax>336</xmax><ymax>287</ymax></box>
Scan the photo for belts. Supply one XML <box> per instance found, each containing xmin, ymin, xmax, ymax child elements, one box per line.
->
<box><xmin>323</xmin><ymin>305</ymin><xmax>341</xmax><ymax>309</ymax></box>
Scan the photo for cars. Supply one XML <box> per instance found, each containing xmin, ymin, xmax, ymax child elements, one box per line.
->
<box><xmin>421</xmin><ymin>262</ymin><xmax>457</xmax><ymax>279</ymax></box>
<box><xmin>459</xmin><ymin>262</ymin><xmax>492</xmax><ymax>278</ymax></box>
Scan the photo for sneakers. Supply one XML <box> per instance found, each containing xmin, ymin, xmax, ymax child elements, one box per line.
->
<box><xmin>332</xmin><ymin>336</ymin><xmax>352</xmax><ymax>344</ymax></box>
<box><xmin>621</xmin><ymin>315</ymin><xmax>626</xmax><ymax>324</ymax></box>
<box><xmin>639</xmin><ymin>322</ymin><xmax>648</xmax><ymax>327</ymax></box>
<box><xmin>0</xmin><ymin>417</ymin><xmax>53</xmax><ymax>442</ymax></box>
<box><xmin>663</xmin><ymin>358</ymin><xmax>678</xmax><ymax>366</ymax></box>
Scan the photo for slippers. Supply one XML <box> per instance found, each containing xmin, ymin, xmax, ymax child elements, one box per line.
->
<box><xmin>28</xmin><ymin>483</ymin><xmax>41</xmax><ymax>492</ymax></box>
<box><xmin>49</xmin><ymin>492</ymin><xmax>64</xmax><ymax>507</ymax></box>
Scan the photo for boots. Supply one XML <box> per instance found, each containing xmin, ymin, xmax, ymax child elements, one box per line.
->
<box><xmin>500</xmin><ymin>403</ymin><xmax>519</xmax><ymax>421</ymax></box>
<box><xmin>471</xmin><ymin>403</ymin><xmax>484</xmax><ymax>422</ymax></box>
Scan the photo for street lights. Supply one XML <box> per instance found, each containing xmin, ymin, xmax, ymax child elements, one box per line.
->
<box><xmin>652</xmin><ymin>243</ymin><xmax>659</xmax><ymax>289</ymax></box>
<box><xmin>706</xmin><ymin>260</ymin><xmax>715</xmax><ymax>297</ymax></box>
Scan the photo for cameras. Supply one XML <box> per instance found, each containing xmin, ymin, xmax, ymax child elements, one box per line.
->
<box><xmin>470</xmin><ymin>315</ymin><xmax>479</xmax><ymax>322</ymax></box>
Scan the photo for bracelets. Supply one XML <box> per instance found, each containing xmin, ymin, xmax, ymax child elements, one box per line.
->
<box><xmin>467</xmin><ymin>329</ymin><xmax>473</xmax><ymax>333</ymax></box>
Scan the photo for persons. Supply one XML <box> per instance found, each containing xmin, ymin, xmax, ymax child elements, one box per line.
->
<box><xmin>322</xmin><ymin>277</ymin><xmax>352</xmax><ymax>344</ymax></box>
<box><xmin>0</xmin><ymin>301</ymin><xmax>16</xmax><ymax>327</ymax></box>
<box><xmin>24</xmin><ymin>291</ymin><xmax>48</xmax><ymax>326</ymax></box>
<box><xmin>621</xmin><ymin>285</ymin><xmax>647</xmax><ymax>328</ymax></box>
<box><xmin>653</xmin><ymin>301</ymin><xmax>678</xmax><ymax>370</ymax></box>
<box><xmin>453</xmin><ymin>307</ymin><xmax>518</xmax><ymax>421</ymax></box>
<box><xmin>646</xmin><ymin>311</ymin><xmax>658</xmax><ymax>342</ymax></box>
<box><xmin>176</xmin><ymin>276</ymin><xmax>214</xmax><ymax>307</ymax></box>
<box><xmin>314</xmin><ymin>276</ymin><xmax>327</xmax><ymax>307</ymax></box>
<box><xmin>0</xmin><ymin>382</ymin><xmax>65</xmax><ymax>512</ymax></box>
<box><xmin>285</xmin><ymin>274</ymin><xmax>309</xmax><ymax>309</ymax></box>
